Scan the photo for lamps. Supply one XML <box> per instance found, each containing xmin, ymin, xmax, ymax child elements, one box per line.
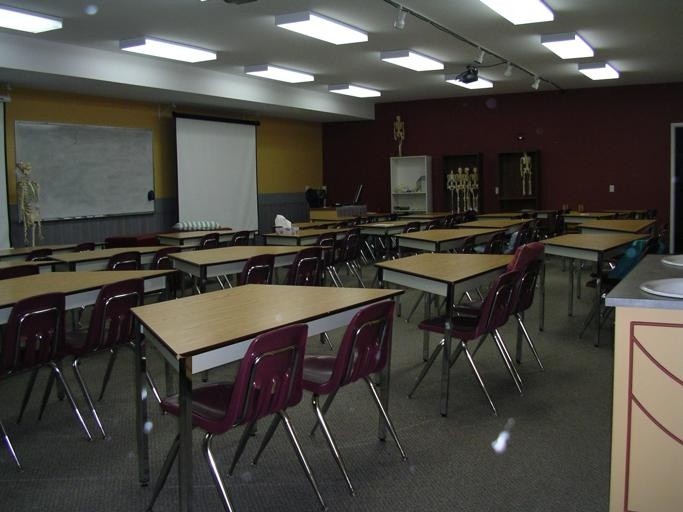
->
<box><xmin>472</xmin><ymin>46</ymin><xmax>485</xmax><ymax>64</ymax></box>
<box><xmin>578</xmin><ymin>62</ymin><xmax>621</xmax><ymax>81</ymax></box>
<box><xmin>118</xmin><ymin>32</ymin><xmax>217</xmax><ymax>63</ymax></box>
<box><xmin>392</xmin><ymin>5</ymin><xmax>408</xmax><ymax>30</ymax></box>
<box><xmin>275</xmin><ymin>9</ymin><xmax>369</xmax><ymax>46</ymax></box>
<box><xmin>244</xmin><ymin>63</ymin><xmax>315</xmax><ymax>84</ymax></box>
<box><xmin>480</xmin><ymin>0</ymin><xmax>556</xmax><ymax>26</ymax></box>
<box><xmin>531</xmin><ymin>75</ymin><xmax>540</xmax><ymax>90</ymax></box>
<box><xmin>504</xmin><ymin>61</ymin><xmax>513</xmax><ymax>78</ymax></box>
<box><xmin>539</xmin><ymin>32</ymin><xmax>595</xmax><ymax>60</ymax></box>
<box><xmin>441</xmin><ymin>73</ymin><xmax>496</xmax><ymax>93</ymax></box>
<box><xmin>327</xmin><ymin>83</ymin><xmax>381</xmax><ymax>98</ymax></box>
<box><xmin>380</xmin><ymin>49</ymin><xmax>444</xmax><ymax>72</ymax></box>
<box><xmin>0</xmin><ymin>5</ymin><xmax>63</xmax><ymax>34</ymax></box>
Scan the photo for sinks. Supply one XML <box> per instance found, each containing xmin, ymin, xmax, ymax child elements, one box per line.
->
<box><xmin>640</xmin><ymin>276</ymin><xmax>683</xmax><ymax>301</ymax></box>
<box><xmin>659</xmin><ymin>253</ymin><xmax>683</xmax><ymax>268</ymax></box>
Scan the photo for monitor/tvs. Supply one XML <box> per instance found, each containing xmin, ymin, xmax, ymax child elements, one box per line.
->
<box><xmin>352</xmin><ymin>184</ymin><xmax>363</xmax><ymax>205</ymax></box>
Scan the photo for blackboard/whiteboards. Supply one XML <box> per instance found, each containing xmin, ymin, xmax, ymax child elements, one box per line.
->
<box><xmin>14</xmin><ymin>120</ymin><xmax>156</xmax><ymax>223</ymax></box>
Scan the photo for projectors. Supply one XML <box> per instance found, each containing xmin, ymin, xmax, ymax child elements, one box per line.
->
<box><xmin>455</xmin><ymin>64</ymin><xmax>479</xmax><ymax>85</ymax></box>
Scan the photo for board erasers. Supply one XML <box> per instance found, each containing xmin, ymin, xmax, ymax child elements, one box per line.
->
<box><xmin>148</xmin><ymin>190</ymin><xmax>155</xmax><ymax>202</ymax></box>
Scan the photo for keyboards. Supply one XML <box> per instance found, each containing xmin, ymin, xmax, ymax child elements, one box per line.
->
<box><xmin>331</xmin><ymin>201</ymin><xmax>351</xmax><ymax>207</ymax></box>
